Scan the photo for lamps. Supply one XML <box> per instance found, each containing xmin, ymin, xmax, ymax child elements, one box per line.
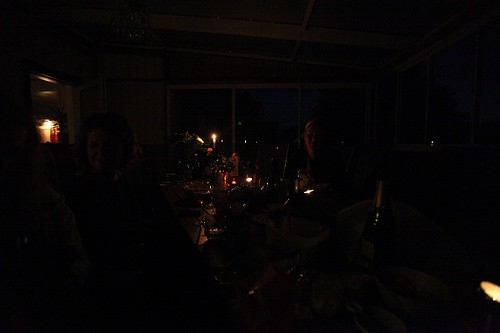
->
<box><xmin>110</xmin><ymin>0</ymin><xmax>149</xmax><ymax>44</ymax></box>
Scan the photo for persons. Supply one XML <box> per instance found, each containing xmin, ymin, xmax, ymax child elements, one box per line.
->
<box><xmin>63</xmin><ymin>111</ymin><xmax>163</xmax><ymax>282</ymax></box>
<box><xmin>286</xmin><ymin>120</ymin><xmax>343</xmax><ymax>183</ymax></box>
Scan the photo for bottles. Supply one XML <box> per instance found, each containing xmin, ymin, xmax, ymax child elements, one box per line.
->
<box><xmin>357</xmin><ymin>175</ymin><xmax>399</xmax><ymax>277</ymax></box>
